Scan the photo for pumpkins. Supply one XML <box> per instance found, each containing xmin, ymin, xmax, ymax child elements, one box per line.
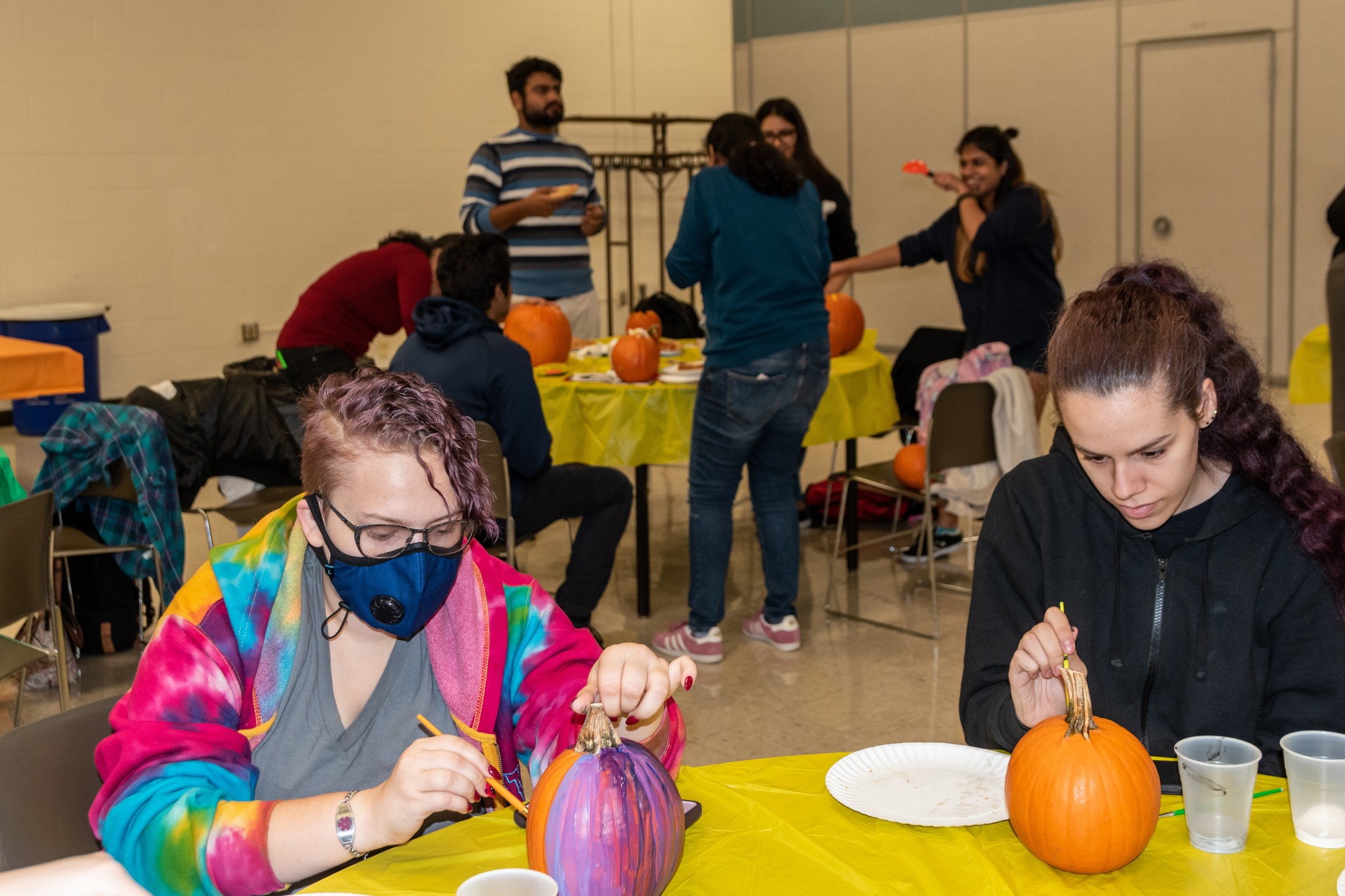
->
<box><xmin>499</xmin><ymin>292</ymin><xmax>866</xmax><ymax>384</ymax></box>
<box><xmin>526</xmin><ymin>703</ymin><xmax>685</xmax><ymax>896</ymax></box>
<box><xmin>1006</xmin><ymin>666</ymin><xmax>1161</xmax><ymax>875</ymax></box>
<box><xmin>895</xmin><ymin>446</ymin><xmax>926</xmax><ymax>490</ymax></box>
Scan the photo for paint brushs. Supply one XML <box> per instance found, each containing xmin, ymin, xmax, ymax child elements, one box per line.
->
<box><xmin>1060</xmin><ymin>601</ymin><xmax>1070</xmax><ymax>715</ymax></box>
<box><xmin>1156</xmin><ymin>788</ymin><xmax>1283</xmax><ymax>822</ymax></box>
<box><xmin>415</xmin><ymin>713</ymin><xmax>529</xmax><ymax>818</ymax></box>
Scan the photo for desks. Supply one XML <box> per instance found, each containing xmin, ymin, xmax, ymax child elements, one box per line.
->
<box><xmin>528</xmin><ymin>333</ymin><xmax>902</xmax><ymax>619</ymax></box>
<box><xmin>0</xmin><ymin>335</ymin><xmax>86</xmax><ymax>403</ymax></box>
<box><xmin>273</xmin><ymin>752</ymin><xmax>1345</xmax><ymax>896</ymax></box>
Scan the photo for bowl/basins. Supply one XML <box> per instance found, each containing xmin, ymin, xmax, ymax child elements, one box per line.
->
<box><xmin>456</xmin><ymin>868</ymin><xmax>558</xmax><ymax>896</ymax></box>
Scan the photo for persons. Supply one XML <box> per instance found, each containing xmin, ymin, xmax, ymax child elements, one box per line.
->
<box><xmin>829</xmin><ymin>123</ymin><xmax>1067</xmax><ymax>563</ymax></box>
<box><xmin>461</xmin><ymin>56</ymin><xmax>609</xmax><ymax>342</ymax></box>
<box><xmin>87</xmin><ymin>380</ymin><xmax>700</xmax><ymax>895</ymax></box>
<box><xmin>648</xmin><ymin>111</ymin><xmax>831</xmax><ymax>665</ymax></box>
<box><xmin>957</xmin><ymin>257</ymin><xmax>1344</xmax><ymax>778</ymax></box>
<box><xmin>387</xmin><ymin>233</ymin><xmax>635</xmax><ymax>627</ymax></box>
<box><xmin>755</xmin><ymin>97</ymin><xmax>858</xmax><ymax>294</ymax></box>
<box><xmin>276</xmin><ymin>231</ymin><xmax>463</xmax><ymax>398</ymax></box>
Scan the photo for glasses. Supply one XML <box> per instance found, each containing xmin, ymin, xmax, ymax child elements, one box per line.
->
<box><xmin>313</xmin><ymin>489</ymin><xmax>480</xmax><ymax>560</ymax></box>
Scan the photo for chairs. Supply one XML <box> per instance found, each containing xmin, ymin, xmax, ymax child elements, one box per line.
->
<box><xmin>31</xmin><ymin>402</ymin><xmax>167</xmax><ymax>686</ymax></box>
<box><xmin>151</xmin><ymin>371</ymin><xmax>306</xmax><ymax>549</ymax></box>
<box><xmin>824</xmin><ymin>379</ymin><xmax>998</xmax><ymax>641</ymax></box>
<box><xmin>816</xmin><ymin>324</ymin><xmax>1030</xmax><ymax>538</ymax></box>
<box><xmin>466</xmin><ymin>416</ymin><xmax>574</xmax><ymax>568</ymax></box>
<box><xmin>0</xmin><ymin>488</ymin><xmax>67</xmax><ymax>728</ymax></box>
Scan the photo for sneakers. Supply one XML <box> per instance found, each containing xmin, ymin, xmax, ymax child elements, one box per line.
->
<box><xmin>652</xmin><ymin>610</ymin><xmax>724</xmax><ymax>663</ymax></box>
<box><xmin>896</xmin><ymin>523</ymin><xmax>964</xmax><ymax>562</ymax></box>
<box><xmin>742</xmin><ymin>611</ymin><xmax>801</xmax><ymax>651</ymax></box>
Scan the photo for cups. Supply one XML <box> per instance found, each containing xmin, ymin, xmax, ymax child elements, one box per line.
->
<box><xmin>1172</xmin><ymin>734</ymin><xmax>1263</xmax><ymax>854</ymax></box>
<box><xmin>1280</xmin><ymin>731</ymin><xmax>1345</xmax><ymax>849</ymax></box>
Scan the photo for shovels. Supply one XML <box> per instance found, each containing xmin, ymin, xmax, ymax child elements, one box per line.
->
<box><xmin>901</xmin><ymin>158</ymin><xmax>933</xmax><ymax>178</ymax></box>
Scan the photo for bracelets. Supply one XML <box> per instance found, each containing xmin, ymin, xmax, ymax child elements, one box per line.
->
<box><xmin>336</xmin><ymin>789</ymin><xmax>368</xmax><ymax>860</ymax></box>
<box><xmin>957</xmin><ymin>192</ymin><xmax>976</xmax><ymax>205</ymax></box>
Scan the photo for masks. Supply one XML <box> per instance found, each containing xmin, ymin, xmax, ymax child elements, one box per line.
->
<box><xmin>302</xmin><ymin>494</ymin><xmax>466</xmax><ymax>639</ymax></box>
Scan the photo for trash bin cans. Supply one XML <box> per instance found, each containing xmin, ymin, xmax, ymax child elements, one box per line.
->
<box><xmin>0</xmin><ymin>301</ymin><xmax>111</xmax><ymax>437</ymax></box>
<box><xmin>227</xmin><ymin>354</ymin><xmax>277</xmax><ymax>543</ymax></box>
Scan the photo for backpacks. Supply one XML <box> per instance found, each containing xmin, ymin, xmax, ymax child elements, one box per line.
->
<box><xmin>45</xmin><ymin>496</ymin><xmax>154</xmax><ymax>657</ymax></box>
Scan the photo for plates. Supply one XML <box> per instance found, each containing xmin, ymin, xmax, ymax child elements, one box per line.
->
<box><xmin>659</xmin><ymin>361</ymin><xmax>704</xmax><ymax>382</ymax></box>
<box><xmin>825</xmin><ymin>741</ymin><xmax>1008</xmax><ymax>828</ymax></box>
